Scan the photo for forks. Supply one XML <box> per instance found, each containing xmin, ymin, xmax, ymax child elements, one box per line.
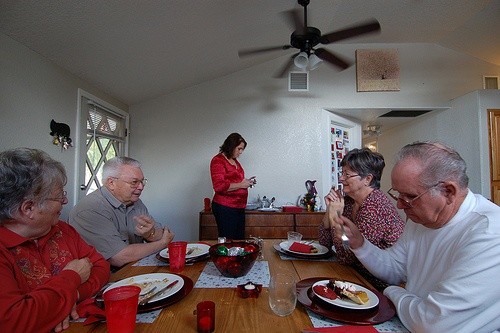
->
<box><xmin>139</xmin><ymin>286</ymin><xmax>156</xmax><ymax>296</ymax></box>
<box><xmin>185</xmin><ymin>248</ymin><xmax>193</xmax><ymax>255</ymax></box>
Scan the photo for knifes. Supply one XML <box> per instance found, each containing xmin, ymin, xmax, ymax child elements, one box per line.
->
<box><xmin>140</xmin><ymin>280</ymin><xmax>179</xmax><ymax>305</ymax></box>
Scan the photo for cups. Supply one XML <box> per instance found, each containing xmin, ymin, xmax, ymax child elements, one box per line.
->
<box><xmin>196</xmin><ymin>301</ymin><xmax>215</xmax><ymax>333</ymax></box>
<box><xmin>167</xmin><ymin>241</ymin><xmax>187</xmax><ymax>273</ymax></box>
<box><xmin>204</xmin><ymin>197</ymin><xmax>210</xmax><ymax>211</ymax></box>
<box><xmin>287</xmin><ymin>231</ymin><xmax>302</xmax><ymax>241</ymax></box>
<box><xmin>269</xmin><ymin>272</ymin><xmax>295</xmax><ymax>317</ymax></box>
<box><xmin>102</xmin><ymin>285</ymin><xmax>141</xmax><ymax>333</ymax></box>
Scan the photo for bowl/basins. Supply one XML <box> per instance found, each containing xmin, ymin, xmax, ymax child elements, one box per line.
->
<box><xmin>245</xmin><ymin>204</ymin><xmax>257</xmax><ymax>210</ymax></box>
<box><xmin>208</xmin><ymin>242</ymin><xmax>258</xmax><ymax>278</ymax></box>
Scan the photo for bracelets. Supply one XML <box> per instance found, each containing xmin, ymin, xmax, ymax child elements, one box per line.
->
<box><xmin>145</xmin><ymin>229</ymin><xmax>155</xmax><ymax>240</ymax></box>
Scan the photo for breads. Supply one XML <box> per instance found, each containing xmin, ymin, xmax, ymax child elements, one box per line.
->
<box><xmin>343</xmin><ymin>290</ymin><xmax>364</xmax><ymax>304</ymax></box>
<box><xmin>354</xmin><ymin>291</ymin><xmax>369</xmax><ymax>302</ymax></box>
<box><xmin>310</xmin><ymin>247</ymin><xmax>319</xmax><ymax>253</ymax></box>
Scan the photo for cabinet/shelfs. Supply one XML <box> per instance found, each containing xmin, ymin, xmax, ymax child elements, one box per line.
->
<box><xmin>199</xmin><ymin>209</ymin><xmax>327</xmax><ymax>241</ymax></box>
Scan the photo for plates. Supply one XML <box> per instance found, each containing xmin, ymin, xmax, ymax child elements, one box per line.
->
<box><xmin>156</xmin><ymin>241</ymin><xmax>214</xmax><ymax>264</ymax></box>
<box><xmin>94</xmin><ymin>273</ymin><xmax>194</xmax><ymax>312</ymax></box>
<box><xmin>258</xmin><ymin>208</ymin><xmax>278</xmax><ymax>212</ymax></box>
<box><xmin>312</xmin><ymin>280</ymin><xmax>380</xmax><ymax>310</ymax></box>
<box><xmin>275</xmin><ymin>242</ymin><xmax>336</xmax><ymax>258</ymax></box>
<box><xmin>159</xmin><ymin>243</ymin><xmax>210</xmax><ymax>259</ymax></box>
<box><xmin>295</xmin><ymin>277</ymin><xmax>397</xmax><ymax>324</ymax></box>
<box><xmin>280</xmin><ymin>240</ymin><xmax>328</xmax><ymax>255</ymax></box>
<box><xmin>102</xmin><ymin>273</ymin><xmax>184</xmax><ymax>304</ymax></box>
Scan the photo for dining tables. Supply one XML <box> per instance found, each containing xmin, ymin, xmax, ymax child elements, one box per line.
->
<box><xmin>50</xmin><ymin>238</ymin><xmax>408</xmax><ymax>333</ymax></box>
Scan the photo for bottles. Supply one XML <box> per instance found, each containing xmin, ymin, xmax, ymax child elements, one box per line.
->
<box><xmin>255</xmin><ymin>236</ymin><xmax>264</xmax><ymax>260</ymax></box>
<box><xmin>257</xmin><ymin>194</ymin><xmax>275</xmax><ymax>208</ymax></box>
<box><xmin>246</xmin><ymin>236</ymin><xmax>257</xmax><ymax>243</ymax></box>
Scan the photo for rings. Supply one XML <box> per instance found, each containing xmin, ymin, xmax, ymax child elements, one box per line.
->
<box><xmin>140</xmin><ymin>226</ymin><xmax>143</xmax><ymax>229</ymax></box>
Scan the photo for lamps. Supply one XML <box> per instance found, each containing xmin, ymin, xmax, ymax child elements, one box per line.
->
<box><xmin>294</xmin><ymin>0</ymin><xmax>323</xmax><ymax>71</ymax></box>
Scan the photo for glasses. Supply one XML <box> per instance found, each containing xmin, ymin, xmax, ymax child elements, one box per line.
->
<box><xmin>387</xmin><ymin>181</ymin><xmax>445</xmax><ymax>208</ymax></box>
<box><xmin>110</xmin><ymin>176</ymin><xmax>147</xmax><ymax>186</ymax></box>
<box><xmin>45</xmin><ymin>191</ymin><xmax>67</xmax><ymax>205</ymax></box>
<box><xmin>339</xmin><ymin>171</ymin><xmax>360</xmax><ymax>180</ymax></box>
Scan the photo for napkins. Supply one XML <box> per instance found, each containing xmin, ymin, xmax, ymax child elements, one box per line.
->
<box><xmin>74</xmin><ymin>297</ymin><xmax>106</xmax><ymax>326</ymax></box>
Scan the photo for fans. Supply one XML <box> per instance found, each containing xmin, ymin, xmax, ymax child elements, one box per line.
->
<box><xmin>236</xmin><ymin>0</ymin><xmax>382</xmax><ymax>79</ymax></box>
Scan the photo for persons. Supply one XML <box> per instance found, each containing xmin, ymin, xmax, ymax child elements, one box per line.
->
<box><xmin>334</xmin><ymin>143</ymin><xmax>500</xmax><ymax>333</ymax></box>
<box><xmin>318</xmin><ymin>147</ymin><xmax>405</xmax><ymax>290</ymax></box>
<box><xmin>69</xmin><ymin>157</ymin><xmax>174</xmax><ymax>273</ymax></box>
<box><xmin>0</xmin><ymin>148</ymin><xmax>110</xmax><ymax>333</ymax></box>
<box><xmin>210</xmin><ymin>133</ymin><xmax>256</xmax><ymax>240</ymax></box>
